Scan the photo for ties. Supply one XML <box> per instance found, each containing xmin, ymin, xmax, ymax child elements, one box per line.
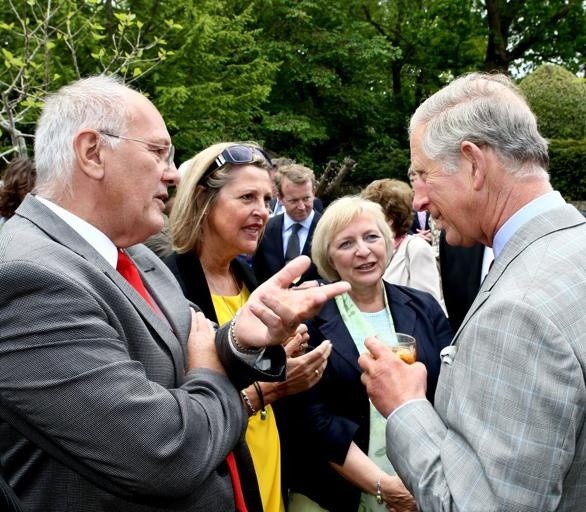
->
<box><xmin>116</xmin><ymin>247</ymin><xmax>246</xmax><ymax>508</ymax></box>
<box><xmin>285</xmin><ymin>224</ymin><xmax>301</xmax><ymax>261</ymax></box>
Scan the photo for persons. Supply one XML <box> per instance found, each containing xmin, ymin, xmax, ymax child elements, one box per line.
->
<box><xmin>357</xmin><ymin>69</ymin><xmax>586</xmax><ymax>512</ymax></box>
<box><xmin>0</xmin><ymin>156</ymin><xmax>34</xmax><ymax>227</ymax></box>
<box><xmin>441</xmin><ymin>226</ymin><xmax>494</xmax><ymax>334</ymax></box>
<box><xmin>407</xmin><ymin>165</ymin><xmax>434</xmax><ymax>247</ymax></box>
<box><xmin>160</xmin><ymin>140</ymin><xmax>335</xmax><ymax>512</ymax></box>
<box><xmin>267</xmin><ymin>157</ymin><xmax>322</xmax><ymax>213</ymax></box>
<box><xmin>0</xmin><ymin>72</ymin><xmax>352</xmax><ymax>512</ymax></box>
<box><xmin>253</xmin><ymin>164</ymin><xmax>326</xmax><ymax>287</ymax></box>
<box><xmin>290</xmin><ymin>193</ymin><xmax>454</xmax><ymax>512</ymax></box>
<box><xmin>362</xmin><ymin>178</ymin><xmax>453</xmax><ymax>320</ymax></box>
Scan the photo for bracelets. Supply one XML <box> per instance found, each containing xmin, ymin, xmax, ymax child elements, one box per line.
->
<box><xmin>375</xmin><ymin>473</ymin><xmax>384</xmax><ymax>504</ymax></box>
<box><xmin>237</xmin><ymin>388</ymin><xmax>255</xmax><ymax>416</ymax></box>
<box><xmin>250</xmin><ymin>380</ymin><xmax>266</xmax><ymax>420</ymax></box>
<box><xmin>230</xmin><ymin>310</ymin><xmax>263</xmax><ymax>356</ymax></box>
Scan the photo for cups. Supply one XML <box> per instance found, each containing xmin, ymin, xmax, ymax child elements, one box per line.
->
<box><xmin>372</xmin><ymin>330</ymin><xmax>417</xmax><ymax>366</ymax></box>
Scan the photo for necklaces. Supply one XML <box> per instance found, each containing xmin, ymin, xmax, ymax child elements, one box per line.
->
<box><xmin>208</xmin><ymin>267</ymin><xmax>243</xmax><ymax>317</ymax></box>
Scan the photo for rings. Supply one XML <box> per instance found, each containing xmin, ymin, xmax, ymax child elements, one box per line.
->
<box><xmin>298</xmin><ymin>343</ymin><xmax>304</xmax><ymax>351</ymax></box>
<box><xmin>314</xmin><ymin>369</ymin><xmax>320</xmax><ymax>376</ymax></box>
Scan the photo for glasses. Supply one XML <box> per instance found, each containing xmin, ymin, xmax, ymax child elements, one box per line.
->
<box><xmin>101</xmin><ymin>129</ymin><xmax>177</xmax><ymax>166</ymax></box>
<box><xmin>200</xmin><ymin>145</ymin><xmax>273</xmax><ymax>184</ymax></box>
